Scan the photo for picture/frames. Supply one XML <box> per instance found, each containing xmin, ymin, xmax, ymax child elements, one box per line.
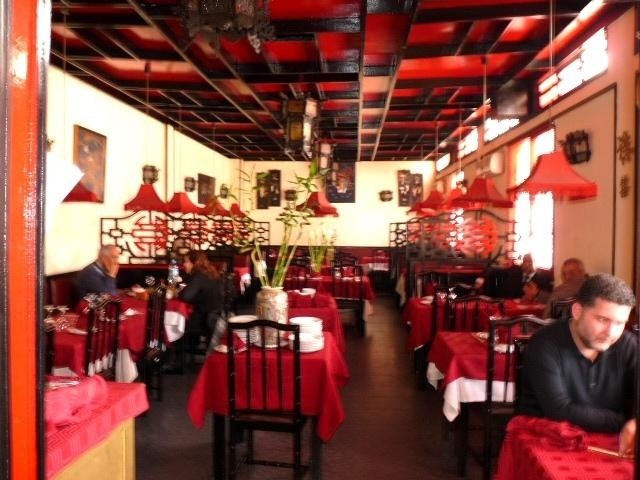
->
<box><xmin>70</xmin><ymin>125</ymin><xmax>106</xmax><ymax>203</ymax></box>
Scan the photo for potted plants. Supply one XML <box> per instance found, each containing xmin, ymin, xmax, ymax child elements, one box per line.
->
<box><xmin>222</xmin><ymin>161</ymin><xmax>343</xmax><ymax>346</ymax></box>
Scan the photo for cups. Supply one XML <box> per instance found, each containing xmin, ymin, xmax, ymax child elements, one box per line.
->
<box><xmin>145</xmin><ymin>275</ymin><xmax>155</xmax><ymax>286</ymax></box>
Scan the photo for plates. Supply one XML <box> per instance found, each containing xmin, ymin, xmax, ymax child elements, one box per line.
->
<box><xmin>227</xmin><ymin>314</ymin><xmax>259</xmax><ymax>344</ymax></box>
<box><xmin>286</xmin><ymin>316</ymin><xmax>325</xmax><ymax>354</ymax></box>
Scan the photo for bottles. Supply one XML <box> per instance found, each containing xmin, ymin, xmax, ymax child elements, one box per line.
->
<box><xmin>168</xmin><ymin>259</ymin><xmax>180</xmax><ymax>289</ymax></box>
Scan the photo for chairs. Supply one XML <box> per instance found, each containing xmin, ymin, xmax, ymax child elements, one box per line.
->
<box><xmin>40</xmin><ymin>267</ymin><xmax>195</xmax><ymax>402</ymax></box>
<box><xmin>196</xmin><ymin>247</ymin><xmax>364</xmax><ymax>479</ymax></box>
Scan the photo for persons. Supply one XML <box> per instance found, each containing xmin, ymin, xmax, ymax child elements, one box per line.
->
<box><xmin>165</xmin><ymin>249</ymin><xmax>226</xmax><ymax>375</ymax></box>
<box><xmin>541</xmin><ymin>257</ymin><xmax>588</xmax><ymax>318</ymax></box>
<box><xmin>72</xmin><ymin>245</ymin><xmax>121</xmax><ymax>308</ymax></box>
<box><xmin>473</xmin><ymin>252</ymin><xmax>550</xmax><ymax>302</ymax></box>
<box><xmin>510</xmin><ymin>271</ymin><xmax>638</xmax><ymax>457</ymax></box>
